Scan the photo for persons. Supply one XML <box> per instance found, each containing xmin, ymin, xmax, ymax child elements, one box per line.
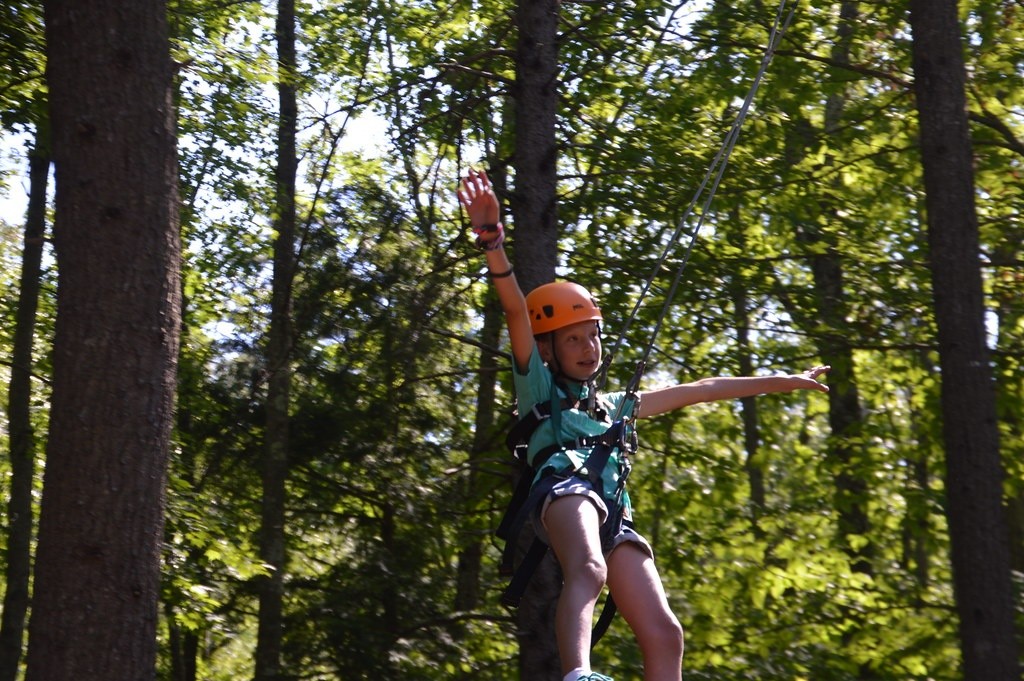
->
<box><xmin>455</xmin><ymin>166</ymin><xmax>831</xmax><ymax>681</ymax></box>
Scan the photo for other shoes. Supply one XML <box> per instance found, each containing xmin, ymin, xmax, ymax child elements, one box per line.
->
<box><xmin>577</xmin><ymin>672</ymin><xmax>613</xmax><ymax>681</ymax></box>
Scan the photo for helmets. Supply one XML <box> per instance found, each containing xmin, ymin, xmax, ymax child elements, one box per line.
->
<box><xmin>525</xmin><ymin>282</ymin><xmax>604</xmax><ymax>335</ymax></box>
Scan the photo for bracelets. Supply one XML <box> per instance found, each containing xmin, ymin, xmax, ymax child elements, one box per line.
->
<box><xmin>475</xmin><ymin>231</ymin><xmax>505</xmax><ymax>251</ymax></box>
<box><xmin>472</xmin><ymin>222</ymin><xmax>503</xmax><ymax>234</ymax></box>
<box><xmin>489</xmin><ymin>263</ymin><xmax>513</xmax><ymax>278</ymax></box>
